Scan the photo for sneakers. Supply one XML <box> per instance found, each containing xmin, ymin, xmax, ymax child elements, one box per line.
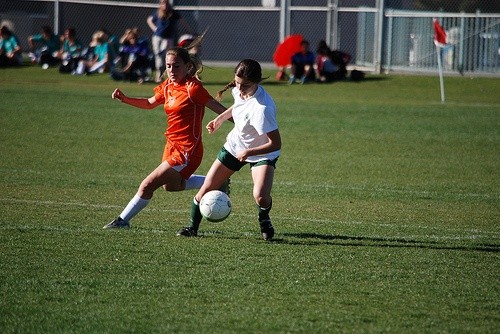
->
<box><xmin>176</xmin><ymin>226</ymin><xmax>197</xmax><ymax>237</ymax></box>
<box><xmin>220</xmin><ymin>177</ymin><xmax>231</xmax><ymax>197</ymax></box>
<box><xmin>257</xmin><ymin>215</ymin><xmax>274</xmax><ymax>240</ymax></box>
<box><xmin>102</xmin><ymin>215</ymin><xmax>130</xmax><ymax>229</ymax></box>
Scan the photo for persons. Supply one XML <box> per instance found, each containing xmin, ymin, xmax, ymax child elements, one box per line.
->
<box><xmin>0</xmin><ymin>20</ymin><xmax>353</xmax><ymax>86</ymax></box>
<box><xmin>145</xmin><ymin>0</ymin><xmax>195</xmax><ymax>83</ymax></box>
<box><xmin>285</xmin><ymin>40</ymin><xmax>315</xmax><ymax>86</ymax></box>
<box><xmin>175</xmin><ymin>58</ymin><xmax>281</xmax><ymax>241</ymax></box>
<box><xmin>101</xmin><ymin>47</ymin><xmax>234</xmax><ymax>230</ymax></box>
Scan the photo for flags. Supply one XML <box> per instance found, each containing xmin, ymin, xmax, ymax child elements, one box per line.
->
<box><xmin>433</xmin><ymin>21</ymin><xmax>447</xmax><ymax>49</ymax></box>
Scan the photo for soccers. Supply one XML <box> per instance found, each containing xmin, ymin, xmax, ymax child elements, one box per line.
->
<box><xmin>199</xmin><ymin>191</ymin><xmax>232</xmax><ymax>223</ymax></box>
